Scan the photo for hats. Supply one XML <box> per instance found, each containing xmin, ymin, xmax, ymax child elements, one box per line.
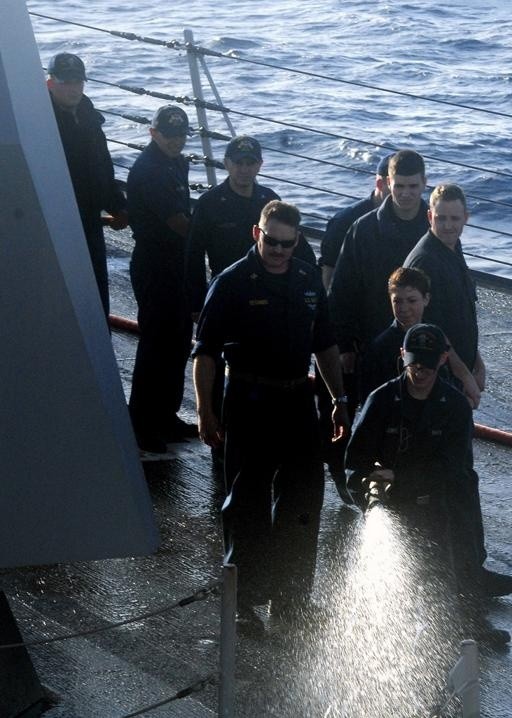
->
<box><xmin>151</xmin><ymin>104</ymin><xmax>194</xmax><ymax>138</ymax></box>
<box><xmin>224</xmin><ymin>136</ymin><xmax>262</xmax><ymax>163</ymax></box>
<box><xmin>403</xmin><ymin>324</ymin><xmax>446</xmax><ymax>370</ymax></box>
<box><xmin>47</xmin><ymin>53</ymin><xmax>88</xmax><ymax>81</ymax></box>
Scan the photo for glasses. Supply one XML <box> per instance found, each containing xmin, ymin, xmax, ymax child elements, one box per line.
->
<box><xmin>258</xmin><ymin>229</ymin><xmax>296</xmax><ymax>247</ymax></box>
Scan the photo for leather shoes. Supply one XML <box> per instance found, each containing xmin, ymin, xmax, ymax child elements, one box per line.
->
<box><xmin>236</xmin><ymin>608</ymin><xmax>265</xmax><ymax>633</ymax></box>
<box><xmin>163</xmin><ymin>415</ymin><xmax>199</xmax><ymax>440</ymax></box>
<box><xmin>271</xmin><ymin>596</ymin><xmax>327</xmax><ymax>627</ymax></box>
<box><xmin>475</xmin><ymin>620</ymin><xmax>510</xmax><ymax>645</ymax></box>
<box><xmin>139</xmin><ymin>432</ymin><xmax>168</xmax><ymax>454</ymax></box>
<box><xmin>477</xmin><ymin>566</ymin><xmax>512</xmax><ymax>595</ymax></box>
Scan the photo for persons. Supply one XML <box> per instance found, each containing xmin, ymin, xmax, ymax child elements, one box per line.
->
<box><xmin>325</xmin><ymin>149</ymin><xmax>430</xmax><ymax>505</ymax></box>
<box><xmin>316</xmin><ymin>151</ymin><xmax>399</xmax><ymax>294</ymax></box>
<box><xmin>124</xmin><ymin>105</ymin><xmax>198</xmax><ymax>454</ymax></box>
<box><xmin>45</xmin><ymin>53</ymin><xmax>130</xmax><ymax>332</ymax></box>
<box><xmin>181</xmin><ymin>135</ymin><xmax>314</xmax><ymax>323</ymax></box>
<box><xmin>190</xmin><ymin>200</ymin><xmax>351</xmax><ymax>634</ymax></box>
<box><xmin>342</xmin><ymin>324</ymin><xmax>473</xmax><ymax>645</ymax></box>
<box><xmin>350</xmin><ymin>266</ymin><xmax>511</xmax><ymax>599</ymax></box>
<box><xmin>400</xmin><ymin>182</ymin><xmax>485</xmax><ymax>411</ymax></box>
